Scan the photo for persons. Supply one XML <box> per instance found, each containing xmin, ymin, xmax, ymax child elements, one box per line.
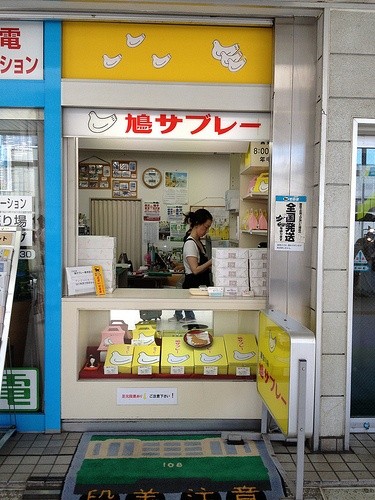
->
<box><xmin>183</xmin><ymin>209</ymin><xmax>213</xmax><ymax>289</ymax></box>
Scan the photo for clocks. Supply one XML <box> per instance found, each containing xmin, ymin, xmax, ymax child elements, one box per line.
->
<box><xmin>142</xmin><ymin>168</ymin><xmax>162</xmax><ymax>187</ymax></box>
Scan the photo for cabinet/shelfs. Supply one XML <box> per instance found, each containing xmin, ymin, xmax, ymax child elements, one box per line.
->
<box><xmin>228</xmin><ymin>153</ymin><xmax>239</xmax><ymax>247</ymax></box>
<box><xmin>239</xmin><ymin>140</ymin><xmax>270</xmax><ymax>246</ymax></box>
<box><xmin>61</xmin><ymin>287</ymin><xmax>268</xmax><ymax>420</ymax></box>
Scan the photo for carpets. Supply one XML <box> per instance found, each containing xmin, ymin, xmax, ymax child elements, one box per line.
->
<box><xmin>61</xmin><ymin>431</ymin><xmax>286</xmax><ymax>500</ymax></box>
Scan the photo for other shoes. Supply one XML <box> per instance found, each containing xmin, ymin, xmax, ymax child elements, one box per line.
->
<box><xmin>180</xmin><ymin>318</ymin><xmax>196</xmax><ymax>323</ymax></box>
<box><xmin>168</xmin><ymin>314</ymin><xmax>180</xmax><ymax>322</ymax></box>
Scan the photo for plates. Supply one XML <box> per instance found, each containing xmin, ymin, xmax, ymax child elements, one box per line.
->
<box><xmin>184</xmin><ymin>330</ymin><xmax>213</xmax><ymax>348</ymax></box>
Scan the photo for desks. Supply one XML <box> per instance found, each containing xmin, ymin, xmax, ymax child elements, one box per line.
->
<box><xmin>130</xmin><ymin>274</ymin><xmax>169</xmax><ymax>287</ymax></box>
<box><xmin>116</xmin><ymin>266</ymin><xmax>128</xmax><ymax>287</ymax></box>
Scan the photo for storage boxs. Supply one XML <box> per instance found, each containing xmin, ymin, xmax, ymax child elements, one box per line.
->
<box><xmin>212</xmin><ymin>248</ymin><xmax>268</xmax><ymax>296</ymax></box>
<box><xmin>103</xmin><ymin>334</ymin><xmax>259</xmax><ymax>374</ymax></box>
<box><xmin>225</xmin><ymin>190</ymin><xmax>239</xmax><ymax>210</ymax></box>
<box><xmin>79</xmin><ymin>235</ymin><xmax>118</xmax><ymax>292</ymax></box>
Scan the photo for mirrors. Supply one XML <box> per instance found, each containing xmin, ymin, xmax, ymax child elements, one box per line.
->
<box><xmin>109</xmin><ymin>310</ymin><xmax>214</xmax><ymax>331</ymax></box>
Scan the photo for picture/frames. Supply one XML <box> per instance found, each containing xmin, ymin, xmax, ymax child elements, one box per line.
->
<box><xmin>79</xmin><ymin>159</ymin><xmax>138</xmax><ymax>198</ymax></box>
